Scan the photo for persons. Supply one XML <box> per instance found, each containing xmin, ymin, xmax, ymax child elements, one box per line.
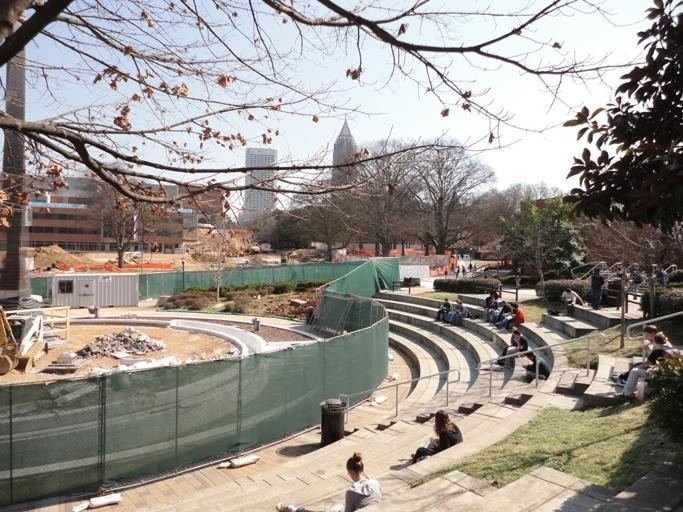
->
<box><xmin>443</xmin><ymin>262</ymin><xmax>478</xmax><ymax>279</ymax></box>
<box><xmin>497</xmin><ymin>326</ymin><xmax>518</xmax><ymax>367</ymax></box>
<box><xmin>655</xmin><ymin>264</ymin><xmax>668</xmax><ymax>290</ymax></box>
<box><xmin>612</xmin><ymin>333</ymin><xmax>674</xmax><ymax>402</ymax></box>
<box><xmin>561</xmin><ymin>287</ymin><xmax>585</xmax><ymax>318</ymax></box>
<box><xmin>615</xmin><ymin>267</ymin><xmax>629</xmax><ymax>289</ymax></box>
<box><xmin>607</xmin><ymin>324</ymin><xmax>672</xmax><ymax>386</ymax></box>
<box><xmin>482</xmin><ymin>291</ymin><xmax>524</xmax><ymax>334</ymax></box>
<box><xmin>629</xmin><ymin>263</ymin><xmax>642</xmax><ymax>301</ymax></box>
<box><xmin>411</xmin><ymin>408</ymin><xmax>464</xmax><ymax>465</ymax></box>
<box><xmin>523</xmin><ymin>351</ymin><xmax>549</xmax><ymax>384</ymax></box>
<box><xmin>434</xmin><ymin>297</ymin><xmax>470</xmax><ymax>329</ymax></box>
<box><xmin>274</xmin><ymin>449</ymin><xmax>382</xmax><ymax>512</ymax></box>
<box><xmin>589</xmin><ymin>268</ymin><xmax>606</xmax><ymax>310</ymax></box>
<box><xmin>499</xmin><ymin>330</ymin><xmax>529</xmax><ymax>370</ymax></box>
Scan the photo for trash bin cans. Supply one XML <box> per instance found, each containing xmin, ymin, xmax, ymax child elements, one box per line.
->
<box><xmin>608</xmin><ymin>278</ymin><xmax>623</xmax><ymax>307</ymax></box>
<box><xmin>320</xmin><ymin>399</ymin><xmax>347</xmax><ymax>446</ymax></box>
